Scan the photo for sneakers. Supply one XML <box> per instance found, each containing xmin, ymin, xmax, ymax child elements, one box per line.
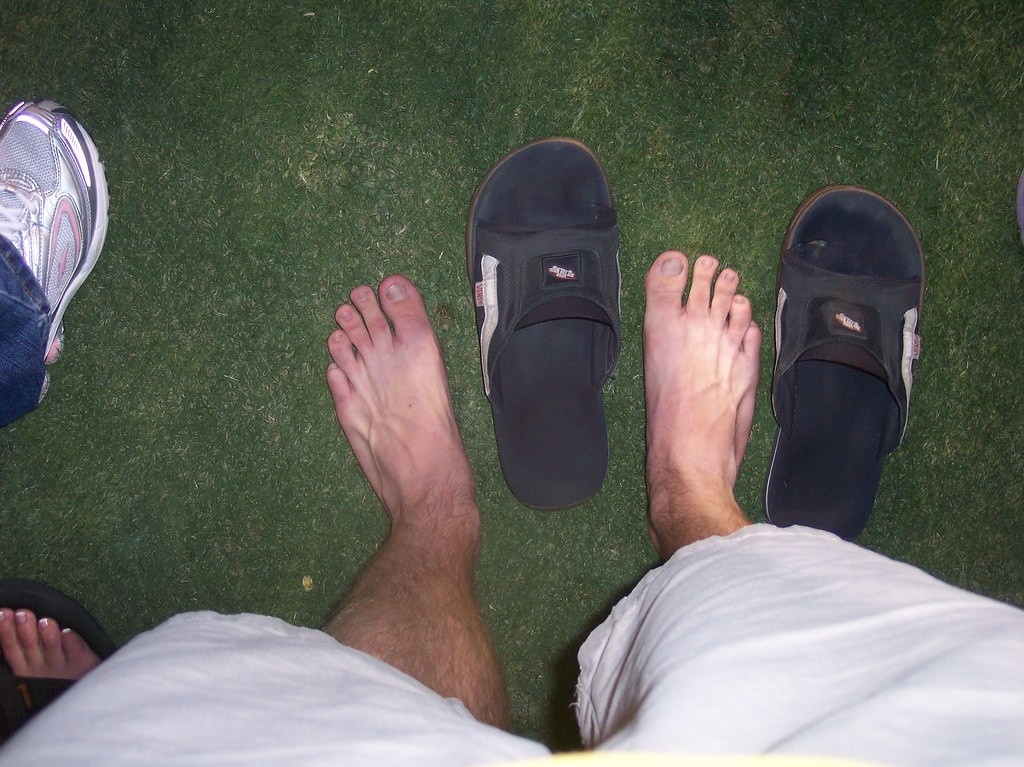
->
<box><xmin>0</xmin><ymin>99</ymin><xmax>110</xmax><ymax>403</ymax></box>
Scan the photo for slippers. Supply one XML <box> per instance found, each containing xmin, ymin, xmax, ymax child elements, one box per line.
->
<box><xmin>466</xmin><ymin>137</ymin><xmax>621</xmax><ymax>514</ymax></box>
<box><xmin>0</xmin><ymin>576</ymin><xmax>117</xmax><ymax>743</ymax></box>
<box><xmin>766</xmin><ymin>185</ymin><xmax>926</xmax><ymax>539</ymax></box>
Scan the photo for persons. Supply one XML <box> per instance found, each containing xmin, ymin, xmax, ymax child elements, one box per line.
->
<box><xmin>0</xmin><ymin>99</ymin><xmax>1024</xmax><ymax>767</ymax></box>
